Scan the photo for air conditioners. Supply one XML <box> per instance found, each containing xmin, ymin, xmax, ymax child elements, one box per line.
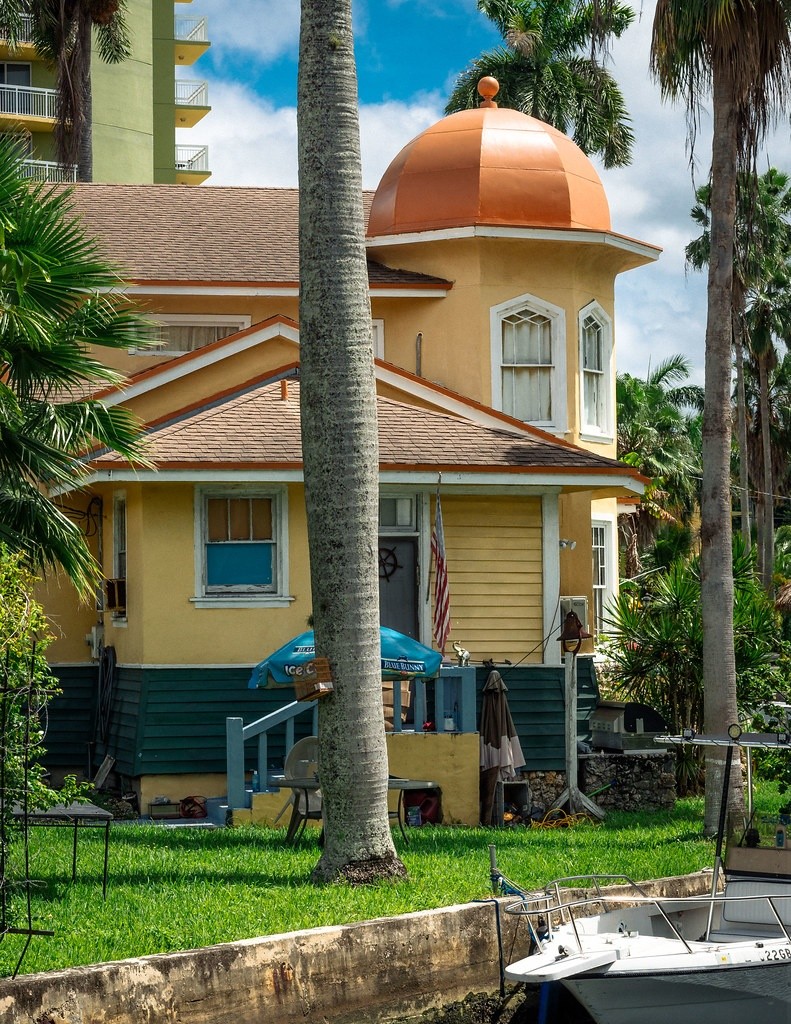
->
<box><xmin>94</xmin><ymin>577</ymin><xmax>125</xmax><ymax>613</ymax></box>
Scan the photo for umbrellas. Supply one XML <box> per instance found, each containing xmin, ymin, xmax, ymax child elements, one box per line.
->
<box><xmin>248</xmin><ymin>626</ymin><xmax>442</xmax><ymax>690</ymax></box>
<box><xmin>480</xmin><ymin>670</ymin><xmax>526</xmax><ymax>827</ymax></box>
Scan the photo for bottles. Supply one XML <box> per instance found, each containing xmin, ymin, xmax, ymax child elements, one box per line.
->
<box><xmin>251</xmin><ymin>770</ymin><xmax>260</xmax><ymax>793</ymax></box>
<box><xmin>775</xmin><ymin>820</ymin><xmax>786</xmax><ymax>850</ymax></box>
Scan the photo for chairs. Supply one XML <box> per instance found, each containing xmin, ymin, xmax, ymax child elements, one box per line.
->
<box><xmin>273</xmin><ymin>735</ymin><xmax>324</xmax><ymax>842</ymax></box>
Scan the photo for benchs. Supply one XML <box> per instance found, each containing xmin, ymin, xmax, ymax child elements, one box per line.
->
<box><xmin>707</xmin><ymin>878</ymin><xmax>791</xmax><ymax>943</ymax></box>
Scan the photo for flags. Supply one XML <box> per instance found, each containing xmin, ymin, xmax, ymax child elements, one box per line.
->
<box><xmin>430</xmin><ymin>487</ymin><xmax>450</xmax><ymax>657</ymax></box>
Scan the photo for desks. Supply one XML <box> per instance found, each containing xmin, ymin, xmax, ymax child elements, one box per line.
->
<box><xmin>1</xmin><ymin>795</ymin><xmax>114</xmax><ymax>903</ymax></box>
<box><xmin>269</xmin><ymin>776</ymin><xmax>437</xmax><ymax>852</ymax></box>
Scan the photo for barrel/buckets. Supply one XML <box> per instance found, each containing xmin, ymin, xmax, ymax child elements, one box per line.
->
<box><xmin>402</xmin><ymin>780</ymin><xmax>443</xmax><ymax>828</ymax></box>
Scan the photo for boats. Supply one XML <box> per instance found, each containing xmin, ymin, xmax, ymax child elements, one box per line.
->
<box><xmin>503</xmin><ymin>723</ymin><xmax>790</xmax><ymax>1024</ymax></box>
